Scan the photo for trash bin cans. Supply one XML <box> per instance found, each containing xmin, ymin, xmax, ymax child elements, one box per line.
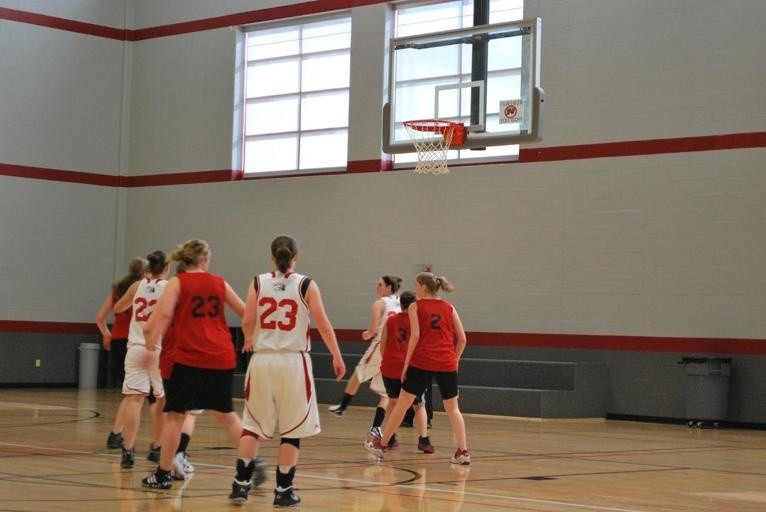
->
<box><xmin>78</xmin><ymin>342</ymin><xmax>101</xmax><ymax>388</ymax></box>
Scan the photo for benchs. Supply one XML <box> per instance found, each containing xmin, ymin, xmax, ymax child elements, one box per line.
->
<box><xmin>231</xmin><ymin>351</ymin><xmax>612</xmax><ymax>419</ymax></box>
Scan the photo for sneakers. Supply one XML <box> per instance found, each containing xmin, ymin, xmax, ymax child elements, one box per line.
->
<box><xmin>253</xmin><ymin>457</ymin><xmax>265</xmax><ymax>485</ymax></box>
<box><xmin>273</xmin><ymin>485</ymin><xmax>300</xmax><ymax>508</ymax></box>
<box><xmin>328</xmin><ymin>405</ymin><xmax>345</xmax><ymax>417</ymax></box>
<box><xmin>400</xmin><ymin>419</ymin><xmax>413</xmax><ymax>427</ymax></box>
<box><xmin>120</xmin><ymin>447</ymin><xmax>134</xmax><ymax>468</ymax></box>
<box><xmin>450</xmin><ymin>448</ymin><xmax>471</xmax><ymax>464</ymax></box>
<box><xmin>148</xmin><ymin>443</ymin><xmax>194</xmax><ymax>479</ymax></box>
<box><xmin>230</xmin><ymin>478</ymin><xmax>252</xmax><ymax>503</ymax></box>
<box><xmin>364</xmin><ymin>426</ymin><xmax>398</xmax><ymax>458</ymax></box>
<box><xmin>418</xmin><ymin>436</ymin><xmax>434</xmax><ymax>453</ymax></box>
<box><xmin>141</xmin><ymin>472</ymin><xmax>172</xmax><ymax>489</ymax></box>
<box><xmin>427</xmin><ymin>418</ymin><xmax>432</xmax><ymax>429</ymax></box>
<box><xmin>106</xmin><ymin>431</ymin><xmax>124</xmax><ymax>448</ymax></box>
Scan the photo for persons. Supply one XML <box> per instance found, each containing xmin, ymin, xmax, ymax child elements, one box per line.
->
<box><xmin>368</xmin><ymin>291</ymin><xmax>435</xmax><ymax>455</ymax></box>
<box><xmin>113</xmin><ymin>251</ymin><xmax>173</xmax><ymax>469</ymax></box>
<box><xmin>96</xmin><ymin>258</ymin><xmax>158</xmax><ymax>449</ymax></box>
<box><xmin>159</xmin><ymin>262</ymin><xmax>204</xmax><ymax>482</ymax></box>
<box><xmin>328</xmin><ymin>274</ymin><xmax>401</xmax><ymax>442</ymax></box>
<box><xmin>364</xmin><ymin>272</ymin><xmax>471</xmax><ymax>465</ymax></box>
<box><xmin>229</xmin><ymin>235</ymin><xmax>347</xmax><ymax>508</ymax></box>
<box><xmin>140</xmin><ymin>239</ymin><xmax>266</xmax><ymax>490</ymax></box>
